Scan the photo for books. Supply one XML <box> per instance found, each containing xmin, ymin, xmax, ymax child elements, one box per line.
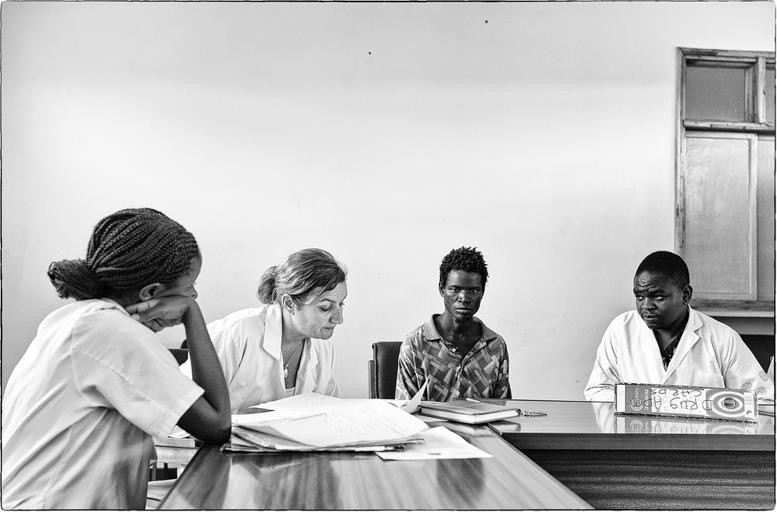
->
<box><xmin>417</xmin><ymin>401</ymin><xmax>521</xmax><ymax>424</ymax></box>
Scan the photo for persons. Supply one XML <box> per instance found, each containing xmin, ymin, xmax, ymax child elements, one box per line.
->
<box><xmin>584</xmin><ymin>251</ymin><xmax>774</xmax><ymax>402</ymax></box>
<box><xmin>395</xmin><ymin>247</ymin><xmax>511</xmax><ymax>400</ymax></box>
<box><xmin>2</xmin><ymin>207</ymin><xmax>232</xmax><ymax>510</ymax></box>
<box><xmin>179</xmin><ymin>248</ymin><xmax>347</xmax><ymax>412</ymax></box>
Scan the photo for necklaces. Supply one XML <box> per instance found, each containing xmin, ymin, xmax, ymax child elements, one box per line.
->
<box><xmin>284</xmin><ymin>341</ymin><xmax>301</xmax><ymax>378</ymax></box>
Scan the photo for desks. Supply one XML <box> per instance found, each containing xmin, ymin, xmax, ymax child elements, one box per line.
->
<box><xmin>158</xmin><ymin>400</ymin><xmax>775</xmax><ymax>509</ymax></box>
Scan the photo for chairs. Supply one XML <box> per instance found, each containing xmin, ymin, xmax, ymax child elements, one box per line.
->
<box><xmin>369</xmin><ymin>341</ymin><xmax>406</xmax><ymax>400</ymax></box>
